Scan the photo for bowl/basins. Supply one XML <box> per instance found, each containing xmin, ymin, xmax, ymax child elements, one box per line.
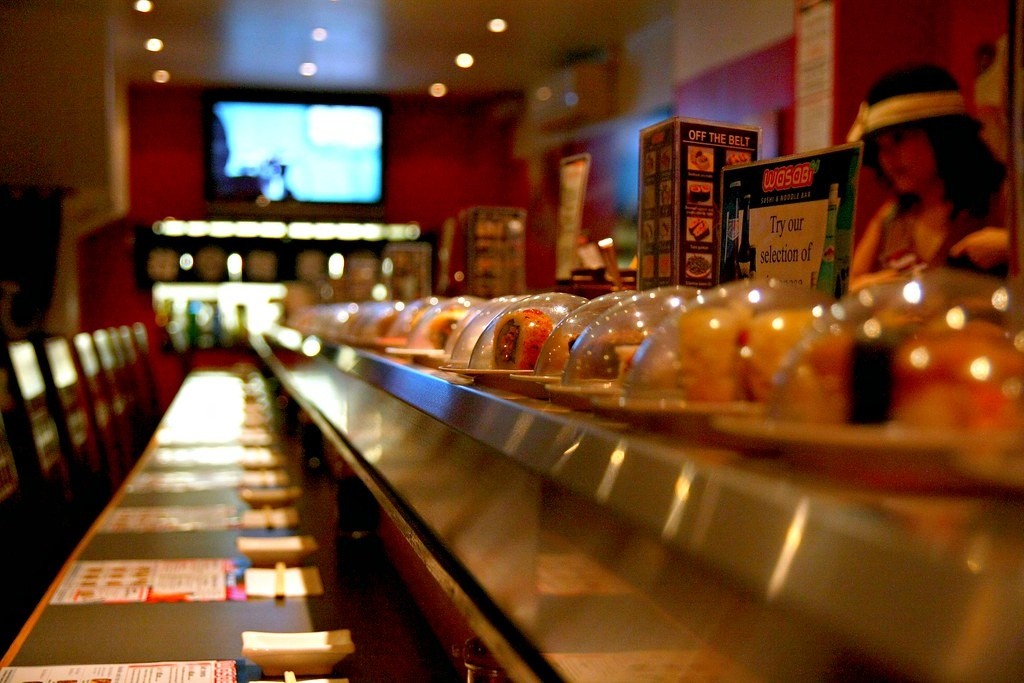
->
<box><xmin>241</xmin><ymin>630</ymin><xmax>354</xmax><ymax>676</ymax></box>
<box><xmin>236</xmin><ymin>372</ymin><xmax>316</xmax><ymax>567</ymax></box>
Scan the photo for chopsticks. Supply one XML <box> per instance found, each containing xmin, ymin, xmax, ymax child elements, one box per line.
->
<box><xmin>261</xmin><ymin>503</ymin><xmax>275</xmax><ymax>529</ymax></box>
<box><xmin>271</xmin><ymin>561</ymin><xmax>286</xmax><ymax>600</ymax></box>
<box><xmin>281</xmin><ymin>670</ymin><xmax>300</xmax><ymax>682</ymax></box>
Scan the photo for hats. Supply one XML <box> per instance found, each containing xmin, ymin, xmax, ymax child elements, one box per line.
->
<box><xmin>846</xmin><ymin>61</ymin><xmax>965</xmax><ymax>143</ymax></box>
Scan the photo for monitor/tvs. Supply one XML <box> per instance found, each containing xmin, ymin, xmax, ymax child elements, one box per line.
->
<box><xmin>199</xmin><ymin>85</ymin><xmax>388</xmax><ymax>208</ymax></box>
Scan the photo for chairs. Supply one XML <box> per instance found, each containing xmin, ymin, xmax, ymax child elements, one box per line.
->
<box><xmin>0</xmin><ymin>322</ymin><xmax>164</xmax><ymax>583</ymax></box>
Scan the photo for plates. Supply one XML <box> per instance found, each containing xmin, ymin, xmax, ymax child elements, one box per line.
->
<box><xmin>284</xmin><ymin>315</ymin><xmax>1024</xmax><ymax>499</ymax></box>
